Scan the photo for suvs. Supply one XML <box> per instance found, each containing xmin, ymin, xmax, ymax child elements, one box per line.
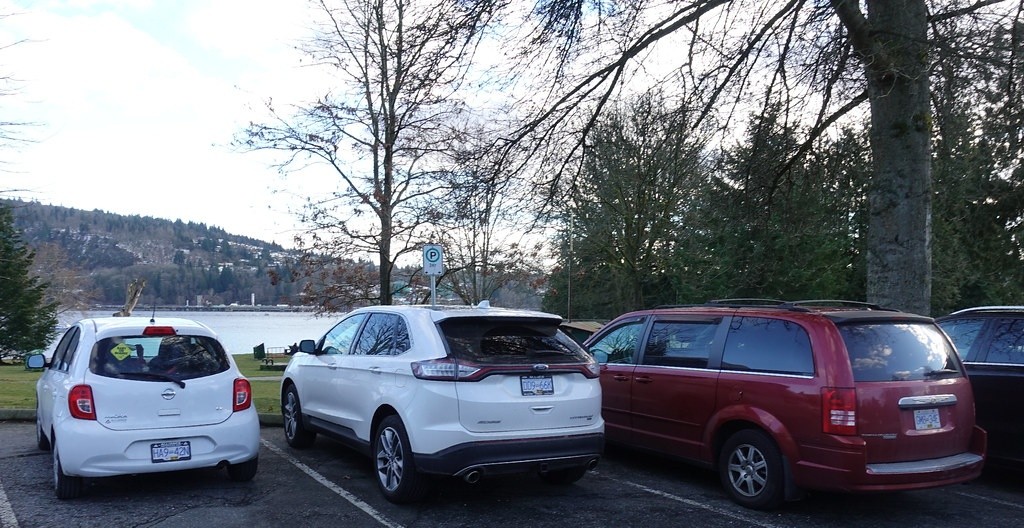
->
<box><xmin>583</xmin><ymin>297</ymin><xmax>990</xmax><ymax>511</ymax></box>
<box><xmin>935</xmin><ymin>304</ymin><xmax>1024</xmax><ymax>475</ymax></box>
<box><xmin>279</xmin><ymin>300</ymin><xmax>606</xmax><ymax>505</ymax></box>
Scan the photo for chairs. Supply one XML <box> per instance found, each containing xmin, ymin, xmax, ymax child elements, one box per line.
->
<box><xmin>149</xmin><ymin>337</ymin><xmax>194</xmax><ymax>372</ymax></box>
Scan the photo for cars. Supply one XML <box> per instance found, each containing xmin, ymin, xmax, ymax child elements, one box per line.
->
<box><xmin>26</xmin><ymin>316</ymin><xmax>262</xmax><ymax>500</ymax></box>
